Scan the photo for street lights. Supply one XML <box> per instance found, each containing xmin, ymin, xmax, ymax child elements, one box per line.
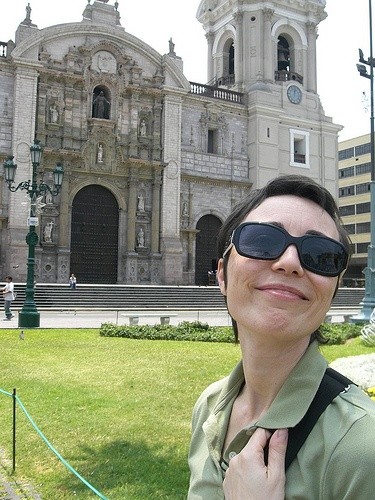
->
<box><xmin>3</xmin><ymin>139</ymin><xmax>65</xmax><ymax>327</ymax></box>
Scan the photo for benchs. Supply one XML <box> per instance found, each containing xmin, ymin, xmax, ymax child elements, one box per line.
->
<box><xmin>121</xmin><ymin>314</ymin><xmax>179</xmax><ymax>326</ymax></box>
<box><xmin>323</xmin><ymin>313</ymin><xmax>358</xmax><ymax>322</ymax></box>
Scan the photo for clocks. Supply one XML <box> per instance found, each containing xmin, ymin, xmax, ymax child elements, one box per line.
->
<box><xmin>287</xmin><ymin>85</ymin><xmax>303</xmax><ymax>104</ymax></box>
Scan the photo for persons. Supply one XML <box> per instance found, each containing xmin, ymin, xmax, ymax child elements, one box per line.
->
<box><xmin>187</xmin><ymin>175</ymin><xmax>375</xmax><ymax>500</ymax></box>
<box><xmin>40</xmin><ymin>90</ymin><xmax>147</xmax><ymax>246</ymax></box>
<box><xmin>208</xmin><ymin>269</ymin><xmax>219</xmax><ymax>285</ymax></box>
<box><xmin>0</xmin><ymin>275</ymin><xmax>15</xmax><ymax>320</ymax></box>
<box><xmin>69</xmin><ymin>274</ymin><xmax>77</xmax><ymax>289</ymax></box>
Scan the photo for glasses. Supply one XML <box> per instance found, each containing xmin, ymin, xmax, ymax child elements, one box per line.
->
<box><xmin>221</xmin><ymin>220</ymin><xmax>351</xmax><ymax>280</ymax></box>
